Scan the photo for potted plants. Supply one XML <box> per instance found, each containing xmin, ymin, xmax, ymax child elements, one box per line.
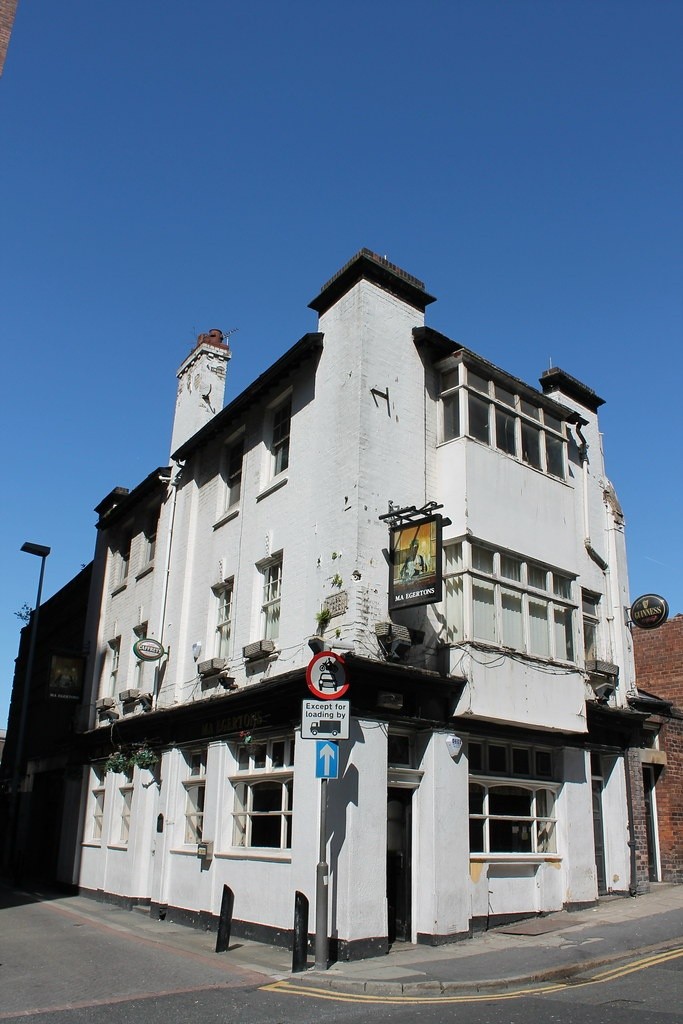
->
<box><xmin>129</xmin><ymin>747</ymin><xmax>160</xmax><ymax>770</ymax></box>
<box><xmin>102</xmin><ymin>751</ymin><xmax>129</xmax><ymax>774</ymax></box>
